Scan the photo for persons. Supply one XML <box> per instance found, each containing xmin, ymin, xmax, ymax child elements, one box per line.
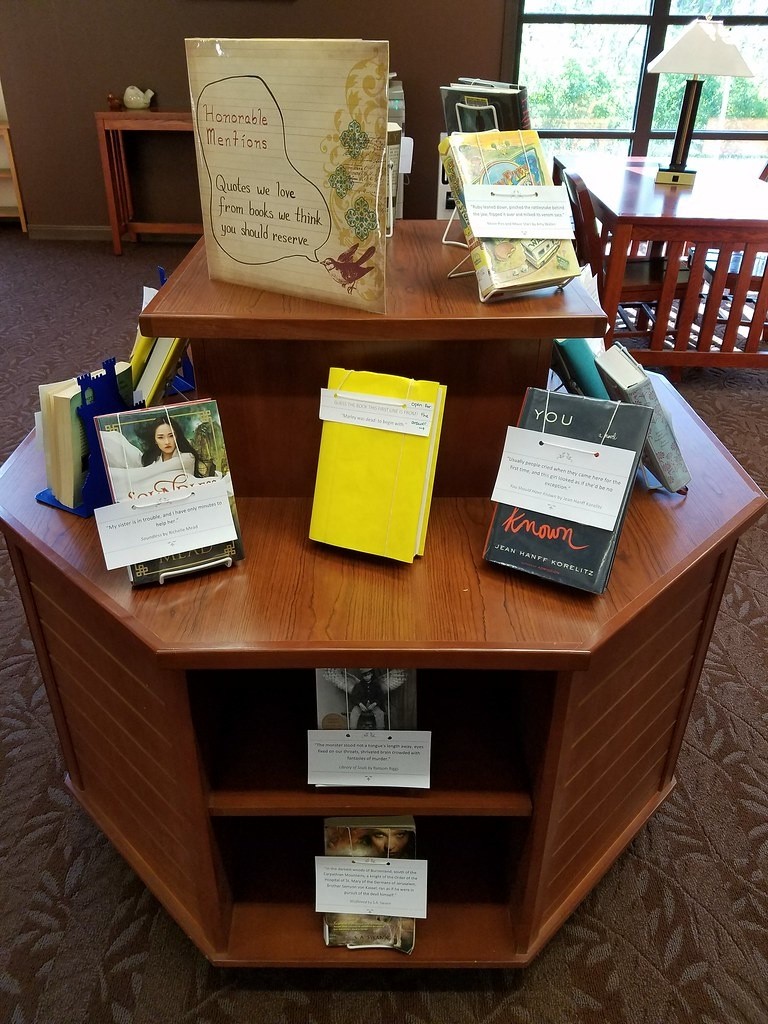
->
<box><xmin>349</xmin><ymin>667</ymin><xmax>386</xmax><ymax>731</ymax></box>
<box><xmin>133</xmin><ymin>410</ymin><xmax>216</xmax><ymax>489</ymax></box>
<box><xmin>324</xmin><ymin>825</ymin><xmax>410</xmax><ymax>859</ymax></box>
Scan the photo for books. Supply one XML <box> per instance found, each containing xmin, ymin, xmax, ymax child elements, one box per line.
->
<box><xmin>305</xmin><ymin>664</ymin><xmax>433</xmax><ymax>791</ymax></box>
<box><xmin>182</xmin><ymin>34</ymin><xmax>393</xmax><ymax>321</ymax></box>
<box><xmin>480</xmin><ymin>385</ymin><xmax>656</xmax><ymax>599</ymax></box>
<box><xmin>308</xmin><ymin>362</ymin><xmax>452</xmax><ymax>567</ymax></box>
<box><xmin>547</xmin><ymin>261</ymin><xmax>694</xmax><ymax>496</ymax></box>
<box><xmin>314</xmin><ymin>813</ymin><xmax>430</xmax><ymax>957</ymax></box>
<box><xmin>35</xmin><ymin>282</ymin><xmax>247</xmax><ymax>594</ymax></box>
<box><xmin>436</xmin><ymin>74</ymin><xmax>583</xmax><ymax>306</ymax></box>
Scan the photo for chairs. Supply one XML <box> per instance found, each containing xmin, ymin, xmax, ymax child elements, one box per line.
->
<box><xmin>555</xmin><ymin>158</ymin><xmax>768</xmax><ymax>385</ymax></box>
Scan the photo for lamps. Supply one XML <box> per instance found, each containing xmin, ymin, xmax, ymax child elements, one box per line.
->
<box><xmin>646</xmin><ymin>11</ymin><xmax>755</xmax><ymax>186</ymax></box>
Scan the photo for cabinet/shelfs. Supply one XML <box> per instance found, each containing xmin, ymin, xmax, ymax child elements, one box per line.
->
<box><xmin>0</xmin><ymin>221</ymin><xmax>768</xmax><ymax>967</ymax></box>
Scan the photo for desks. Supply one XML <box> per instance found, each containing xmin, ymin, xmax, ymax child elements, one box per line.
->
<box><xmin>552</xmin><ymin>153</ymin><xmax>768</xmax><ymax>370</ymax></box>
<box><xmin>0</xmin><ymin>120</ymin><xmax>30</xmax><ymax>237</ymax></box>
<box><xmin>94</xmin><ymin>109</ymin><xmax>206</xmax><ymax>257</ymax></box>
